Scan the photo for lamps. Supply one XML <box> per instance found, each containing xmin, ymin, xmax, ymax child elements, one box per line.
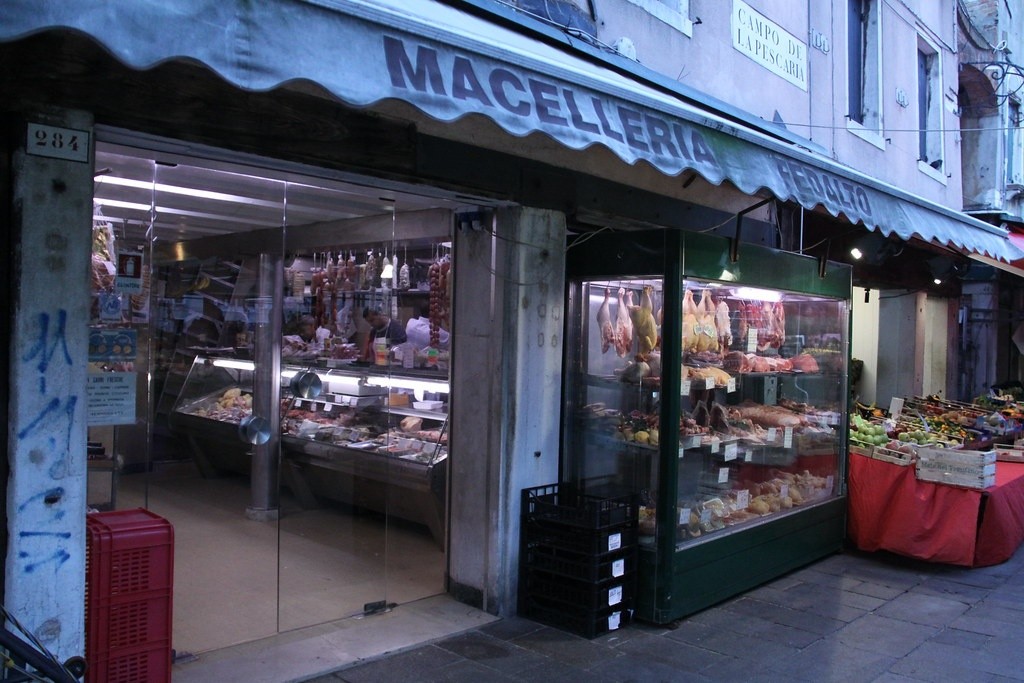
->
<box><xmin>850</xmin><ymin>246</ymin><xmax>868</xmax><ymax>265</ymax></box>
<box><xmin>933</xmin><ymin>264</ymin><xmax>954</xmax><ymax>284</ymax></box>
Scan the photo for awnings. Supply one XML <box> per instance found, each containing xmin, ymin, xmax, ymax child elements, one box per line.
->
<box><xmin>0</xmin><ymin>0</ymin><xmax>1024</xmax><ymax>281</ymax></box>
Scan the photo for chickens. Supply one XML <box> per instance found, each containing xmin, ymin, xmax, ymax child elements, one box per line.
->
<box><xmin>595</xmin><ymin>286</ymin><xmax>732</xmax><ymax>387</ymax></box>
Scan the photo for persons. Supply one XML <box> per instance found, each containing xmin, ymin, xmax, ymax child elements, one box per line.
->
<box><xmin>363</xmin><ymin>304</ymin><xmax>407</xmax><ymax>365</ymax></box>
<box><xmin>406</xmin><ymin>298</ymin><xmax>450</xmax><ymax>371</ymax></box>
<box><xmin>298</xmin><ymin>315</ymin><xmax>338</xmax><ymax>352</ymax></box>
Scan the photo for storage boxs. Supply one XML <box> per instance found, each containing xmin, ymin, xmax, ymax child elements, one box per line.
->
<box><xmin>521</xmin><ymin>481</ymin><xmax>639</xmax><ymax>529</ymax></box>
<box><xmin>518</xmin><ymin>565</ymin><xmax>639</xmax><ymax>611</ymax></box>
<box><xmin>517</xmin><ymin>592</ymin><xmax>638</xmax><ymax>640</ymax></box>
<box><xmin>521</xmin><ymin>513</ymin><xmax>636</xmax><ymax>554</ymax></box>
<box><xmin>872</xmin><ymin>440</ymin><xmax>919</xmax><ymax>466</ymax></box>
<box><xmin>849</xmin><ymin>438</ymin><xmax>873</xmax><ymax>459</ymax></box>
<box><xmin>83</xmin><ymin>506</ymin><xmax>171</xmax><ymax>683</ymax></box>
<box><xmin>519</xmin><ymin>536</ymin><xmax>637</xmax><ymax>582</ymax></box>
<box><xmin>914</xmin><ymin>446</ymin><xmax>996</xmax><ymax>491</ymax></box>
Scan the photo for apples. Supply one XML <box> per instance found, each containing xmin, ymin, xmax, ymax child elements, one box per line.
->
<box><xmin>848</xmin><ymin>414</ymin><xmax>945</xmax><ymax>461</ymax></box>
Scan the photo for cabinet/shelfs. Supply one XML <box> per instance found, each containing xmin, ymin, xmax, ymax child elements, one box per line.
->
<box><xmin>567</xmin><ymin>229</ymin><xmax>853</xmax><ymax>631</ymax></box>
<box><xmin>168</xmin><ymin>236</ymin><xmax>454</xmax><ymax>553</ymax></box>
